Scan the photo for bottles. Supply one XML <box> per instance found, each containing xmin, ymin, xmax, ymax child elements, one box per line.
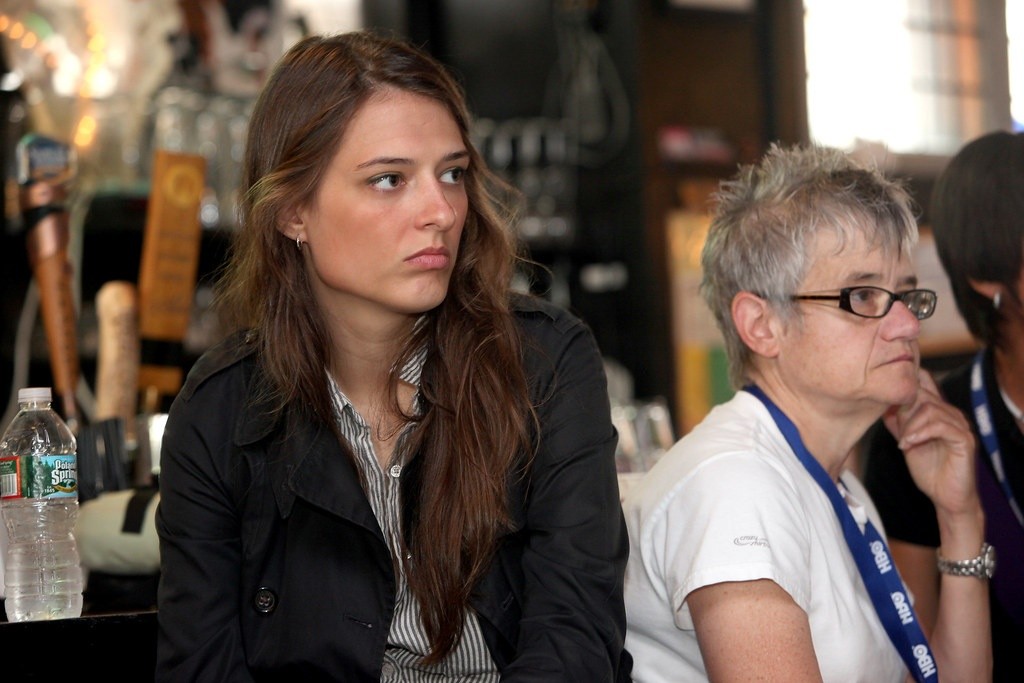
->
<box><xmin>0</xmin><ymin>388</ymin><xmax>85</xmax><ymax>623</ymax></box>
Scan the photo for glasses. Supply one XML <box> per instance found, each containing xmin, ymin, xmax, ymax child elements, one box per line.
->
<box><xmin>790</xmin><ymin>286</ymin><xmax>938</xmax><ymax>320</ymax></box>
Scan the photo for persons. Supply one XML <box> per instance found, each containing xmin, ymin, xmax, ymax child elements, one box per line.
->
<box><xmin>149</xmin><ymin>28</ymin><xmax>636</xmax><ymax>683</ymax></box>
<box><xmin>861</xmin><ymin>131</ymin><xmax>1023</xmax><ymax>682</ymax></box>
<box><xmin>611</xmin><ymin>141</ymin><xmax>994</xmax><ymax>683</ymax></box>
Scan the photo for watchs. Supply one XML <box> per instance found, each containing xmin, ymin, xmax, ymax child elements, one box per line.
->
<box><xmin>937</xmin><ymin>542</ymin><xmax>997</xmax><ymax>580</ymax></box>
